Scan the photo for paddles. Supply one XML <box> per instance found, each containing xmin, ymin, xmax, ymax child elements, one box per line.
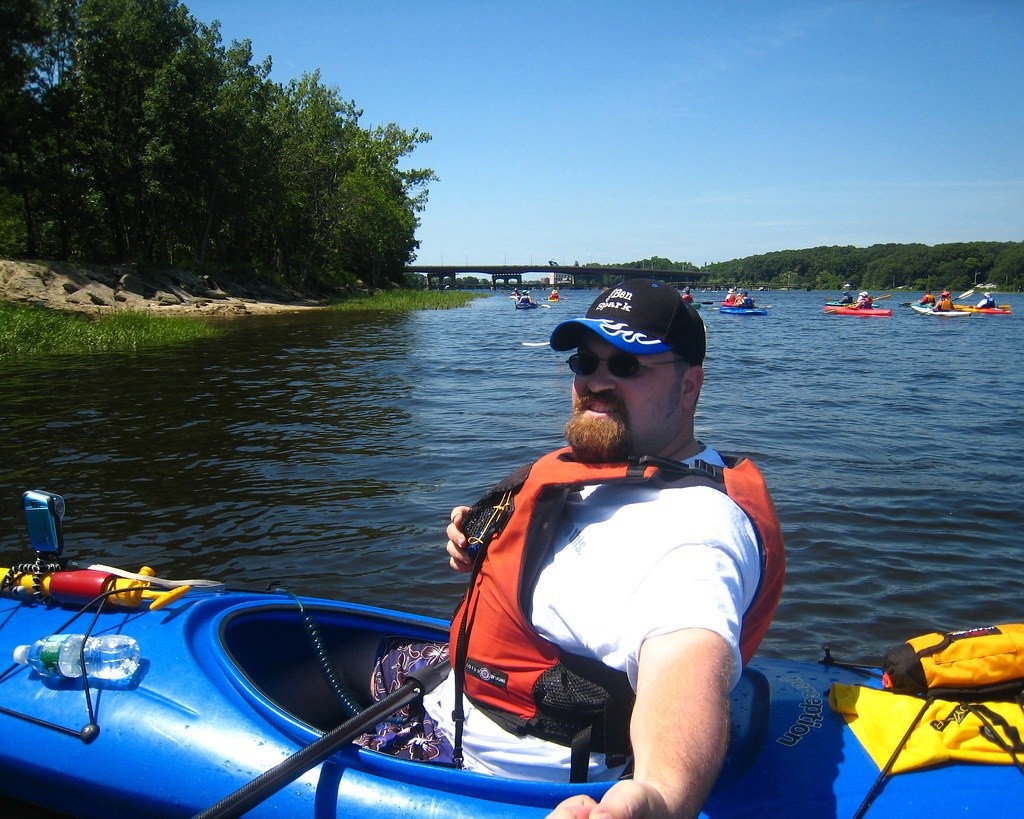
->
<box><xmin>916</xmin><ymin>288</ymin><xmax>975</xmax><ymax>317</ymax></box>
<box><xmin>694</xmin><ymin>300</ymin><xmax>713</xmax><ymax>305</ymax></box>
<box><xmin>192</xmin><ymin>657</ymin><xmax>453</xmax><ymax>819</ymax></box>
<box><xmin>510</xmin><ymin>296</ymin><xmax>551</xmax><ymax>309</ymax></box>
<box><xmin>715</xmin><ymin>299</ymin><xmax>762</xmax><ymax>304</ymax></box>
<box><xmin>954</xmin><ymin>304</ymin><xmax>1011</xmax><ymax>310</ymax></box>
<box><xmin>822</xmin><ymin>293</ymin><xmax>891</xmax><ymax>315</ymax></box>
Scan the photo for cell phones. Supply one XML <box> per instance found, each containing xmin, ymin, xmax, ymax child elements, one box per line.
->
<box><xmin>24</xmin><ymin>492</ymin><xmax>58</xmax><ymax>552</ymax></box>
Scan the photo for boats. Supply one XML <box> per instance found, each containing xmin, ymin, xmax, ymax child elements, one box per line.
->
<box><xmin>822</xmin><ymin>299</ymin><xmax>893</xmax><ymax>317</ymax></box>
<box><xmin>687</xmin><ymin>300</ymin><xmax>771</xmax><ymax>315</ymax></box>
<box><xmin>509</xmin><ymin>291</ymin><xmax>560</xmax><ymax>309</ymax></box>
<box><xmin>0</xmin><ymin>486</ymin><xmax>1024</xmax><ymax>819</ymax></box>
<box><xmin>913</xmin><ymin>300</ymin><xmax>1013</xmax><ymax>317</ymax></box>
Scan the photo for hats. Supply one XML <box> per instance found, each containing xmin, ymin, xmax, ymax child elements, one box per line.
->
<box><xmin>926</xmin><ymin>290</ymin><xmax>931</xmax><ymax>293</ymax></box>
<box><xmin>843</xmin><ymin>291</ymin><xmax>849</xmax><ymax>294</ymax></box>
<box><xmin>727</xmin><ymin>289</ymin><xmax>736</xmax><ymax>294</ymax></box>
<box><xmin>740</xmin><ymin>290</ymin><xmax>748</xmax><ymax>295</ymax></box>
<box><xmin>515</xmin><ymin>288</ymin><xmax>518</xmax><ymax>291</ymax></box>
<box><xmin>941</xmin><ymin>292</ymin><xmax>950</xmax><ymax>296</ymax></box>
<box><xmin>552</xmin><ymin>290</ymin><xmax>557</xmax><ymax>294</ymax></box>
<box><xmin>984</xmin><ymin>292</ymin><xmax>991</xmax><ymax>296</ymax></box>
<box><xmin>859</xmin><ymin>291</ymin><xmax>868</xmax><ymax>297</ymax></box>
<box><xmin>550</xmin><ymin>278</ymin><xmax>706</xmax><ymax>363</ymax></box>
<box><xmin>522</xmin><ymin>290</ymin><xmax>530</xmax><ymax>294</ymax></box>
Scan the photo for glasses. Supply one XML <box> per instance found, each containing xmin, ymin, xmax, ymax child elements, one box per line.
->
<box><xmin>741</xmin><ymin>293</ymin><xmax>743</xmax><ymax>294</ymax></box>
<box><xmin>566</xmin><ymin>353</ymin><xmax>675</xmax><ymax>377</ymax></box>
<box><xmin>845</xmin><ymin>293</ymin><xmax>847</xmax><ymax>294</ymax></box>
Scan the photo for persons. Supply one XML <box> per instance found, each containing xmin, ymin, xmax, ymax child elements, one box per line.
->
<box><xmin>352</xmin><ymin>278</ymin><xmax>786</xmax><ymax>819</ymax></box>
<box><xmin>512</xmin><ymin>287</ymin><xmax>995</xmax><ymax>312</ymax></box>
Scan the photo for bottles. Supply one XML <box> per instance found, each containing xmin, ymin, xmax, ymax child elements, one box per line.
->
<box><xmin>13</xmin><ymin>634</ymin><xmax>139</xmax><ymax>679</ymax></box>
